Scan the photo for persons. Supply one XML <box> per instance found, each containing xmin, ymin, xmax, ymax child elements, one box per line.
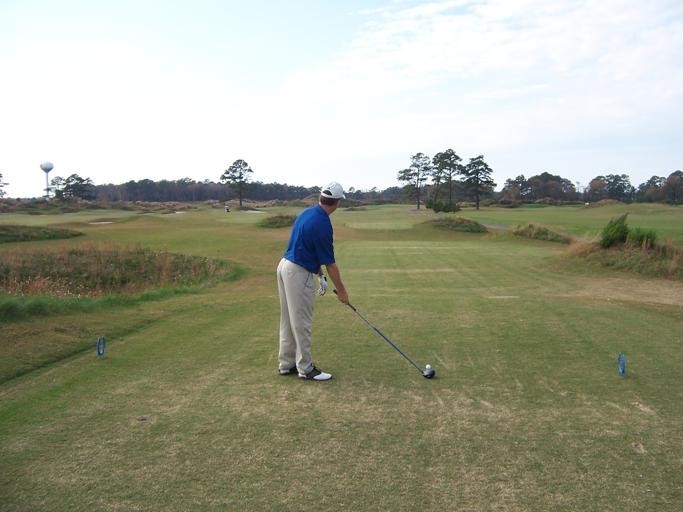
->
<box><xmin>276</xmin><ymin>181</ymin><xmax>349</xmax><ymax>381</ymax></box>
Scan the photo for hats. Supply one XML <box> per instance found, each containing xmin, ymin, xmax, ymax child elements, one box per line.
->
<box><xmin>320</xmin><ymin>181</ymin><xmax>346</xmax><ymax>200</ymax></box>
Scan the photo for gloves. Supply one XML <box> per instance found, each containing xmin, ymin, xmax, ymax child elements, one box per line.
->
<box><xmin>317</xmin><ymin>274</ymin><xmax>328</xmax><ymax>297</ymax></box>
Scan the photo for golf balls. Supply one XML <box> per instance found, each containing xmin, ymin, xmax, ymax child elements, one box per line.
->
<box><xmin>426</xmin><ymin>364</ymin><xmax>431</xmax><ymax>369</ymax></box>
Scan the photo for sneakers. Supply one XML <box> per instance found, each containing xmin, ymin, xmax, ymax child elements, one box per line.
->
<box><xmin>278</xmin><ymin>367</ymin><xmax>332</xmax><ymax>380</ymax></box>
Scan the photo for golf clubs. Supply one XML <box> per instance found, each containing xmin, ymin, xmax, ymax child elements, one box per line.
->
<box><xmin>334</xmin><ymin>290</ymin><xmax>435</xmax><ymax>378</ymax></box>
